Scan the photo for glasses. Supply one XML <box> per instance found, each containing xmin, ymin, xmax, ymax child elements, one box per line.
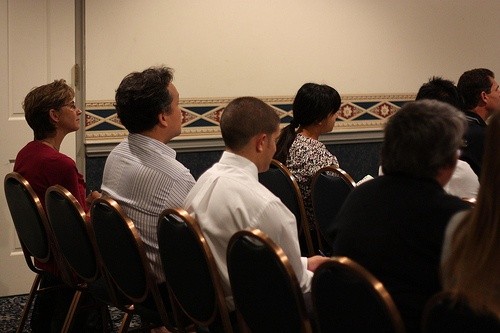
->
<box><xmin>61</xmin><ymin>101</ymin><xmax>76</xmax><ymax>112</ymax></box>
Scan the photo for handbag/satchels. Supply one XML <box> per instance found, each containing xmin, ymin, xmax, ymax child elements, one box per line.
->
<box><xmin>30</xmin><ymin>279</ymin><xmax>113</xmax><ymax>333</ymax></box>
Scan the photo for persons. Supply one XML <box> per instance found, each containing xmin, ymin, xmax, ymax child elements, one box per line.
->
<box><xmin>14</xmin><ymin>78</ymin><xmax>102</xmax><ymax>283</ymax></box>
<box><xmin>336</xmin><ymin>68</ymin><xmax>500</xmax><ymax>333</ymax></box>
<box><xmin>272</xmin><ymin>82</ymin><xmax>342</xmax><ymax>253</ymax></box>
<box><xmin>102</xmin><ymin>68</ymin><xmax>198</xmax><ymax>310</ymax></box>
<box><xmin>185</xmin><ymin>97</ymin><xmax>334</xmax><ymax>320</ymax></box>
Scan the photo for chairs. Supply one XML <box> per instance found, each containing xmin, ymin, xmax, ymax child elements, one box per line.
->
<box><xmin>4</xmin><ymin>159</ymin><xmax>500</xmax><ymax>333</ymax></box>
